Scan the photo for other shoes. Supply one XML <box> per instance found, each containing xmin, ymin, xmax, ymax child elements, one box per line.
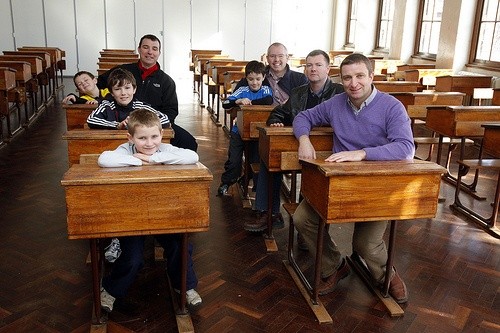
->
<box><xmin>237</xmin><ymin>173</ymin><xmax>253</xmax><ymax>185</ymax></box>
<box><xmin>218</xmin><ymin>183</ymin><xmax>228</xmax><ymax>195</ymax></box>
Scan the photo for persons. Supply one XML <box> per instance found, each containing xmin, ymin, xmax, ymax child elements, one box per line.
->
<box><xmin>98</xmin><ymin>33</ymin><xmax>179</xmax><ymax>123</ymax></box>
<box><xmin>85</xmin><ymin>68</ymin><xmax>172</xmax><ymax>130</ymax></box>
<box><xmin>61</xmin><ymin>70</ymin><xmax>115</xmax><ymax>104</ymax></box>
<box><xmin>97</xmin><ymin>107</ymin><xmax>203</xmax><ymax>313</ymax></box>
<box><xmin>232</xmin><ymin>42</ymin><xmax>310</xmax><ymax>189</ymax></box>
<box><xmin>216</xmin><ymin>59</ymin><xmax>276</xmax><ymax>196</ymax></box>
<box><xmin>292</xmin><ymin>52</ymin><xmax>416</xmax><ymax>302</ymax></box>
<box><xmin>244</xmin><ymin>49</ymin><xmax>347</xmax><ymax>234</ymax></box>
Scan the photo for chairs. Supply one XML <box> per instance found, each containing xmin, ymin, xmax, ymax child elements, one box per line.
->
<box><xmin>203</xmin><ymin>74</ymin><xmax>219</xmax><ymax>93</ymax></box>
<box><xmin>473</xmin><ymin>87</ymin><xmax>494</xmax><ymax>106</ymax></box>
<box><xmin>194</xmin><ymin>71</ymin><xmax>201</xmax><ymax>82</ymax></box>
<box><xmin>0</xmin><ymin>59</ymin><xmax>66</xmax><ymax>149</ymax></box>
<box><xmin>386</xmin><ymin>66</ymin><xmax>398</xmax><ymax>79</ymax></box>
<box><xmin>405</xmin><ymin>103</ymin><xmax>474</xmax><ymax>162</ymax></box>
<box><xmin>84</xmin><ymin>123</ymin><xmax>89</xmax><ymax>129</ymax></box>
<box><xmin>454</xmin><ymin>121</ymin><xmax>500</xmax><ymax>205</ymax></box>
<box><xmin>421</xmin><ymin>76</ymin><xmax>437</xmax><ymax>92</ymax></box>
<box><xmin>220</xmin><ymin>84</ymin><xmax>232</xmax><ymax>99</ymax></box>
<box><xmin>80</xmin><ymin>152</ymin><xmax>187</xmax><ymax>263</ymax></box>
<box><xmin>280</xmin><ymin>150</ymin><xmax>340</xmax><ymax>259</ymax></box>
<box><xmin>394</xmin><ymin>70</ymin><xmax>406</xmax><ymax>80</ymax></box>
<box><xmin>189</xmin><ymin>62</ymin><xmax>195</xmax><ymax>70</ymax></box>
<box><xmin>250</xmin><ymin>121</ymin><xmax>303</xmax><ymax>202</ymax></box>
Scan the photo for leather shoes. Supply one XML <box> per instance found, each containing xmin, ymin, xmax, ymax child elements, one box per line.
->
<box><xmin>388</xmin><ymin>265</ymin><xmax>408</xmax><ymax>304</ymax></box>
<box><xmin>318</xmin><ymin>257</ymin><xmax>351</xmax><ymax>297</ymax></box>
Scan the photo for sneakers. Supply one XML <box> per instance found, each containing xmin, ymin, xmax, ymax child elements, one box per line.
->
<box><xmin>100</xmin><ymin>286</ymin><xmax>116</xmax><ymax>312</ymax></box>
<box><xmin>186</xmin><ymin>288</ymin><xmax>203</xmax><ymax>306</ymax></box>
<box><xmin>242</xmin><ymin>211</ymin><xmax>285</xmax><ymax>232</ymax></box>
<box><xmin>104</xmin><ymin>238</ymin><xmax>122</xmax><ymax>263</ymax></box>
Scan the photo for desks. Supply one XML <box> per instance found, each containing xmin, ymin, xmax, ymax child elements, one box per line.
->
<box><xmin>0</xmin><ymin>45</ymin><xmax>66</xmax><ymax>149</ymax></box>
<box><xmin>63</xmin><ymin>50</ymin><xmax>500</xmax><ymax>333</ymax></box>
